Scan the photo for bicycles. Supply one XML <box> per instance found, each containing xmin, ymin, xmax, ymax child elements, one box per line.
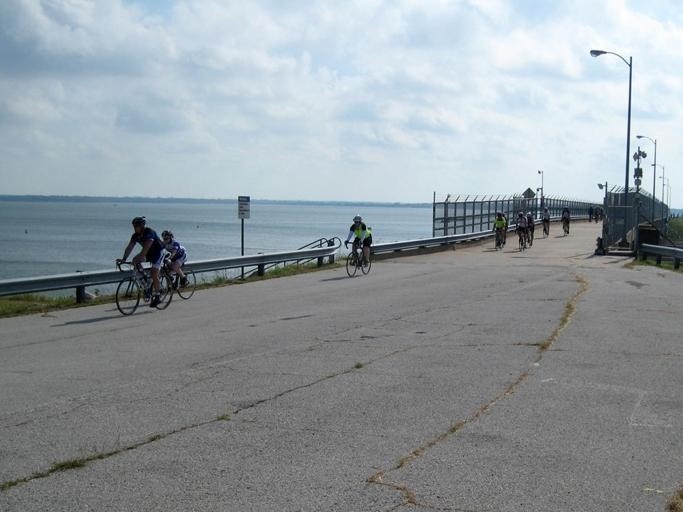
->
<box><xmin>147</xmin><ymin>253</ymin><xmax>197</xmax><ymax>301</ymax></box>
<box><xmin>344</xmin><ymin>240</ymin><xmax>372</xmax><ymax>278</ymax></box>
<box><xmin>111</xmin><ymin>258</ymin><xmax>175</xmax><ymax>317</ymax></box>
<box><xmin>491</xmin><ymin>225</ymin><xmax>533</xmax><ymax>252</ymax></box>
<box><xmin>542</xmin><ymin>219</ymin><xmax>549</xmax><ymax>238</ymax></box>
<box><xmin>587</xmin><ymin>212</ymin><xmax>602</xmax><ymax>223</ymax></box>
<box><xmin>560</xmin><ymin>217</ymin><xmax>569</xmax><ymax>236</ymax></box>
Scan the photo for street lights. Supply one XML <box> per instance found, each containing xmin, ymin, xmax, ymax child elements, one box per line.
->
<box><xmin>647</xmin><ymin>162</ymin><xmax>672</xmax><ymax>222</ymax></box>
<box><xmin>535</xmin><ymin>169</ymin><xmax>543</xmax><ymax>197</ymax></box>
<box><xmin>635</xmin><ymin>134</ymin><xmax>657</xmax><ymax>226</ymax></box>
<box><xmin>596</xmin><ymin>179</ymin><xmax>607</xmax><ymax>207</ymax></box>
<box><xmin>586</xmin><ymin>47</ymin><xmax>632</xmax><ymax>250</ymax></box>
<box><xmin>632</xmin><ymin>145</ymin><xmax>647</xmax><ymax>260</ymax></box>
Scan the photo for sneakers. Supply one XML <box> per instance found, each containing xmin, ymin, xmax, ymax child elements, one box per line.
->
<box><xmin>149</xmin><ymin>295</ymin><xmax>159</xmax><ymax>307</ymax></box>
<box><xmin>363</xmin><ymin>260</ymin><xmax>368</xmax><ymax>267</ymax></box>
<box><xmin>527</xmin><ymin>237</ymin><xmax>533</xmax><ymax>246</ymax></box>
<box><xmin>179</xmin><ymin>277</ymin><xmax>190</xmax><ymax>288</ymax></box>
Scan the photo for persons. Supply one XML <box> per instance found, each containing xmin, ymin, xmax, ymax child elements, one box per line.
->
<box><xmin>345</xmin><ymin>215</ymin><xmax>373</xmax><ymax>267</ymax></box>
<box><xmin>541</xmin><ymin>207</ymin><xmax>550</xmax><ymax>235</ymax></box>
<box><xmin>117</xmin><ymin>217</ymin><xmax>166</xmax><ymax>307</ymax></box>
<box><xmin>493</xmin><ymin>211</ymin><xmax>535</xmax><ymax>246</ymax></box>
<box><xmin>560</xmin><ymin>206</ymin><xmax>569</xmax><ymax>234</ymax></box>
<box><xmin>162</xmin><ymin>230</ymin><xmax>189</xmax><ymax>287</ymax></box>
<box><xmin>588</xmin><ymin>205</ymin><xmax>603</xmax><ymax>223</ymax></box>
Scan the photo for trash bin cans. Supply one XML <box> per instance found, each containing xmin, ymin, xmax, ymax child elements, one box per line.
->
<box><xmin>638</xmin><ymin>224</ymin><xmax>659</xmax><ymax>257</ymax></box>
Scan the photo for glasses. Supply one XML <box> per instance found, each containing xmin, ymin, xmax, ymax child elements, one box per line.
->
<box><xmin>163</xmin><ymin>238</ymin><xmax>171</xmax><ymax>240</ymax></box>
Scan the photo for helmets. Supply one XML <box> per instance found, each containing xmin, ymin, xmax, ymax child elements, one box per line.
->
<box><xmin>162</xmin><ymin>230</ymin><xmax>174</xmax><ymax>238</ymax></box>
<box><xmin>353</xmin><ymin>215</ymin><xmax>361</xmax><ymax>222</ymax></box>
<box><xmin>132</xmin><ymin>216</ymin><xmax>146</xmax><ymax>227</ymax></box>
<box><xmin>494</xmin><ymin>204</ymin><xmax>571</xmax><ymax>218</ymax></box>
<box><xmin>588</xmin><ymin>204</ymin><xmax>603</xmax><ymax>211</ymax></box>
